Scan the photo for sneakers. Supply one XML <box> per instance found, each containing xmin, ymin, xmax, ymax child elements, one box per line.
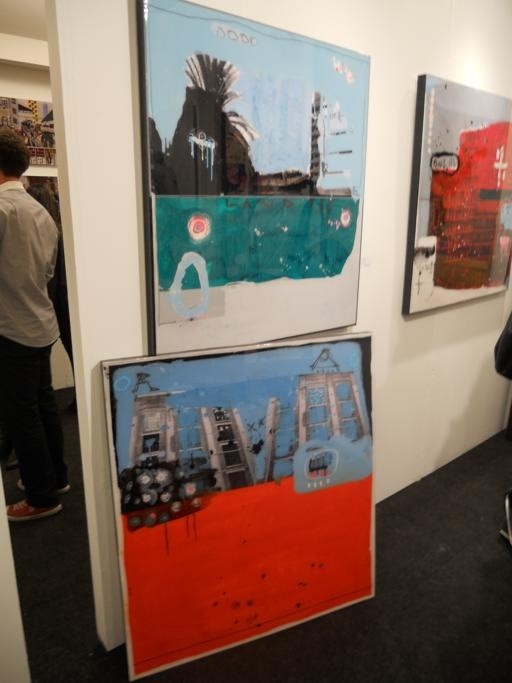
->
<box><xmin>18</xmin><ymin>476</ymin><xmax>70</xmax><ymax>494</ymax></box>
<box><xmin>6</xmin><ymin>500</ymin><xmax>63</xmax><ymax>522</ymax></box>
<box><xmin>5</xmin><ymin>448</ymin><xmax>19</xmax><ymax>467</ymax></box>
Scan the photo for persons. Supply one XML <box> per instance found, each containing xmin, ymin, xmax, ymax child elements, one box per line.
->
<box><xmin>1</xmin><ymin>133</ymin><xmax>74</xmax><ymax>524</ymax></box>
<box><xmin>494</xmin><ymin>307</ymin><xmax>512</xmax><ymax>545</ymax></box>
